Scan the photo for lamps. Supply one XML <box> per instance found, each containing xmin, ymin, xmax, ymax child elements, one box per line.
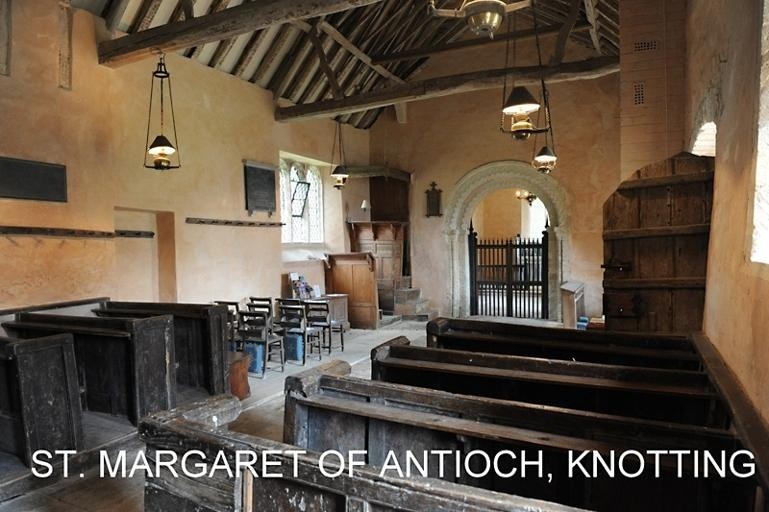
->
<box><xmin>144</xmin><ymin>51</ymin><xmax>182</xmax><ymax>169</ymax></box>
<box><xmin>424</xmin><ymin>0</ymin><xmax>560</xmax><ymax>173</ymax></box>
<box><xmin>329</xmin><ymin>121</ymin><xmax>350</xmax><ymax>189</ymax></box>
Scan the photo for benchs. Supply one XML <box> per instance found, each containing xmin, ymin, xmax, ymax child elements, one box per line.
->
<box><xmin>0</xmin><ymin>300</ymin><xmax>231</xmax><ymax>470</ymax></box>
<box><xmin>139</xmin><ymin>302</ymin><xmax>767</xmax><ymax>512</ymax></box>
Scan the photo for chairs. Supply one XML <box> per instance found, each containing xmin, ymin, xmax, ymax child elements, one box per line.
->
<box><xmin>215</xmin><ymin>297</ymin><xmax>344</xmax><ymax>380</ymax></box>
